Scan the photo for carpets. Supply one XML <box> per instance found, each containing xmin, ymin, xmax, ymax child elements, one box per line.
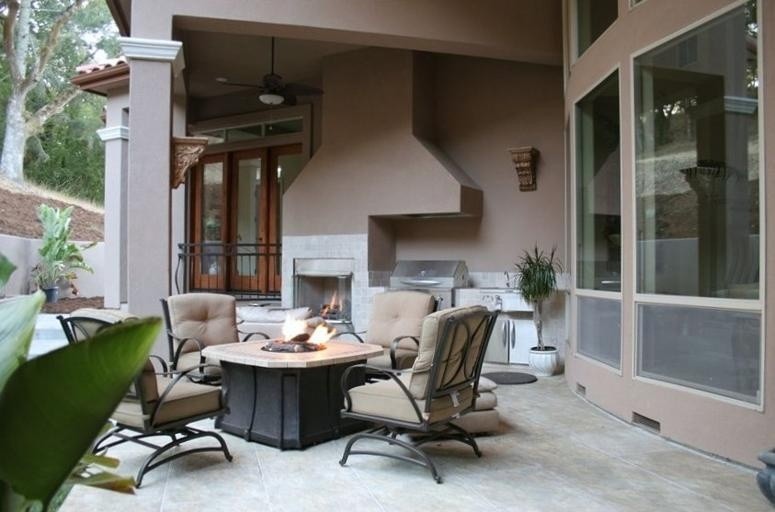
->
<box><xmin>481</xmin><ymin>370</ymin><xmax>539</xmax><ymax>386</ymax></box>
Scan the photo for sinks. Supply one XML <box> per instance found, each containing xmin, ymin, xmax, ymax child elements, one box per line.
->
<box><xmin>480</xmin><ymin>284</ymin><xmax>515</xmax><ymax>292</ymax></box>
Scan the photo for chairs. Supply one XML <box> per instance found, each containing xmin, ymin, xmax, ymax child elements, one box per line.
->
<box><xmin>158</xmin><ymin>290</ymin><xmax>276</xmax><ymax>405</ymax></box>
<box><xmin>333</xmin><ymin>305</ymin><xmax>502</xmax><ymax>484</ymax></box>
<box><xmin>326</xmin><ymin>283</ymin><xmax>446</xmax><ymax>391</ymax></box>
<box><xmin>56</xmin><ymin>306</ymin><xmax>232</xmax><ymax>491</ymax></box>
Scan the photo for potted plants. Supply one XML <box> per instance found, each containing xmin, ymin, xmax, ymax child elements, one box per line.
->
<box><xmin>32</xmin><ymin>202</ymin><xmax>93</xmax><ymax>302</ymax></box>
<box><xmin>513</xmin><ymin>242</ymin><xmax>565</xmax><ymax>379</ymax></box>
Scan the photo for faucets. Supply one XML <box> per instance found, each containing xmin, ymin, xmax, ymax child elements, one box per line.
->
<box><xmin>503</xmin><ymin>270</ymin><xmax>512</xmax><ymax>289</ymax></box>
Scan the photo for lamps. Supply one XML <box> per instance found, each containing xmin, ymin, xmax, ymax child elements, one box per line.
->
<box><xmin>260</xmin><ymin>35</ymin><xmax>286</xmax><ymax>105</ymax></box>
